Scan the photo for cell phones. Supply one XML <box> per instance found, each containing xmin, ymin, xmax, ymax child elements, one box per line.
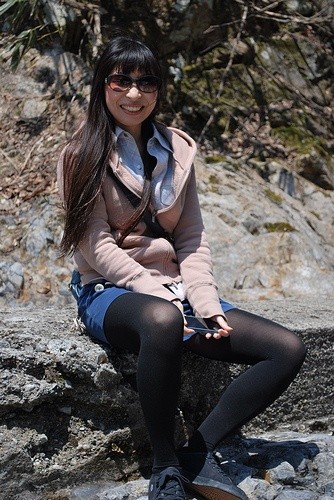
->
<box><xmin>185</xmin><ymin>315</ymin><xmax>222</xmax><ymax>333</ymax></box>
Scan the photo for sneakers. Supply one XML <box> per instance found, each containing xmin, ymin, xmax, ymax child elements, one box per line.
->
<box><xmin>177</xmin><ymin>449</ymin><xmax>248</xmax><ymax>500</ymax></box>
<box><xmin>148</xmin><ymin>466</ymin><xmax>191</xmax><ymax>500</ymax></box>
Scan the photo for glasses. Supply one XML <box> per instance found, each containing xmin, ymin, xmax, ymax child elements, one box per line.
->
<box><xmin>105</xmin><ymin>74</ymin><xmax>164</xmax><ymax>93</ymax></box>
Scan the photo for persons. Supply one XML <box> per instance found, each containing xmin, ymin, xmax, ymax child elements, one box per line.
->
<box><xmin>57</xmin><ymin>37</ymin><xmax>306</xmax><ymax>500</ymax></box>
